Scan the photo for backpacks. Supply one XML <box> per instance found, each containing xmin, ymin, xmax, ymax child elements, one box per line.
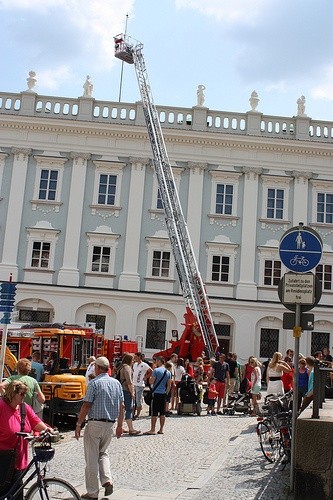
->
<box><xmin>115</xmin><ymin>365</ymin><xmax>126</xmax><ymax>385</ymax></box>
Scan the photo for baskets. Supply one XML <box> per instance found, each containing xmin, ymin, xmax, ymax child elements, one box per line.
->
<box><xmin>267</xmin><ymin>395</ymin><xmax>287</xmax><ymax>413</ymax></box>
<box><xmin>35</xmin><ymin>445</ymin><xmax>55</xmax><ymax>462</ymax></box>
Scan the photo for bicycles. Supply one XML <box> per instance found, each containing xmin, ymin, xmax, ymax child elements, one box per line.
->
<box><xmin>257</xmin><ymin>389</ymin><xmax>293</xmax><ymax>463</ymax></box>
<box><xmin>0</xmin><ymin>427</ymin><xmax>82</xmax><ymax>500</ymax></box>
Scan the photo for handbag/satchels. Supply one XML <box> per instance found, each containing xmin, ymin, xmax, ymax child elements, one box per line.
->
<box><xmin>144</xmin><ymin>390</ymin><xmax>153</xmax><ymax>406</ymax></box>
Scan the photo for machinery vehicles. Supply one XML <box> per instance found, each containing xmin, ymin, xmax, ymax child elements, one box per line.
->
<box><xmin>0</xmin><ymin>345</ymin><xmax>86</xmax><ymax>428</ymax></box>
<box><xmin>0</xmin><ymin>322</ymin><xmax>139</xmax><ymax>420</ymax></box>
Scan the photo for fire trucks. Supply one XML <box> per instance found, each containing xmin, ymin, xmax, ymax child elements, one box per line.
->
<box><xmin>0</xmin><ymin>33</ymin><xmax>221</xmax><ymax>379</ymax></box>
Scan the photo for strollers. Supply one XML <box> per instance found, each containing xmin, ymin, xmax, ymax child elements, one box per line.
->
<box><xmin>223</xmin><ymin>378</ymin><xmax>253</xmax><ymax>417</ymax></box>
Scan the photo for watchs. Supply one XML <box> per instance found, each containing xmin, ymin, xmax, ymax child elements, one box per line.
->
<box><xmin>76</xmin><ymin>422</ymin><xmax>81</xmax><ymax>427</ymax></box>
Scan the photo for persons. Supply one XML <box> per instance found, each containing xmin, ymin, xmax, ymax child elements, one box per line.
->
<box><xmin>0</xmin><ymin>380</ymin><xmax>54</xmax><ymax>500</ymax></box>
<box><xmin>196</xmin><ymin>84</ymin><xmax>206</xmax><ymax>105</ymax></box>
<box><xmin>242</xmin><ymin>346</ymin><xmax>333</xmax><ymax>414</ymax></box>
<box><xmin>26</xmin><ymin>71</ymin><xmax>37</xmax><ymax>89</ymax></box>
<box><xmin>298</xmin><ymin>95</ymin><xmax>306</xmax><ymax>113</ymax></box>
<box><xmin>26</xmin><ymin>352</ymin><xmax>45</xmax><ymax>421</ymax></box>
<box><xmin>83</xmin><ymin>75</ymin><xmax>93</xmax><ymax>95</ymax></box>
<box><xmin>46</xmin><ymin>351</ymin><xmax>69</xmax><ymax>375</ymax></box>
<box><xmin>85</xmin><ymin>352</ymin><xmax>244</xmax><ymax>436</ymax></box>
<box><xmin>248</xmin><ymin>91</ymin><xmax>260</xmax><ymax>109</ymax></box>
<box><xmin>0</xmin><ymin>358</ymin><xmax>45</xmax><ymax>407</ymax></box>
<box><xmin>75</xmin><ymin>356</ymin><xmax>125</xmax><ymax>500</ymax></box>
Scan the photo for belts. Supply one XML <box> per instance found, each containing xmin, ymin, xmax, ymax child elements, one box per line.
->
<box><xmin>88</xmin><ymin>418</ymin><xmax>116</xmax><ymax>423</ymax></box>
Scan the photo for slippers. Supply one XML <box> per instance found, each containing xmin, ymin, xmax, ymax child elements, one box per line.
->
<box><xmin>143</xmin><ymin>431</ymin><xmax>156</xmax><ymax>435</ymax></box>
<box><xmin>157</xmin><ymin>430</ymin><xmax>164</xmax><ymax>434</ymax></box>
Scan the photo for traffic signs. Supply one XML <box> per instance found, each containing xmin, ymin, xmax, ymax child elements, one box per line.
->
<box><xmin>0</xmin><ymin>282</ymin><xmax>18</xmax><ymax>324</ymax></box>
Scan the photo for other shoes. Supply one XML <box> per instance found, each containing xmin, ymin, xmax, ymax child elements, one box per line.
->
<box><xmin>81</xmin><ymin>493</ymin><xmax>98</xmax><ymax>500</ymax></box>
<box><xmin>129</xmin><ymin>430</ymin><xmax>141</xmax><ymax>435</ymax></box>
<box><xmin>104</xmin><ymin>482</ymin><xmax>114</xmax><ymax>497</ymax></box>
<box><xmin>122</xmin><ymin>429</ymin><xmax>129</xmax><ymax>433</ymax></box>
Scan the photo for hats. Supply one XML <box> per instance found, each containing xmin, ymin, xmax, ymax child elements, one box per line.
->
<box><xmin>94</xmin><ymin>358</ymin><xmax>109</xmax><ymax>367</ymax></box>
